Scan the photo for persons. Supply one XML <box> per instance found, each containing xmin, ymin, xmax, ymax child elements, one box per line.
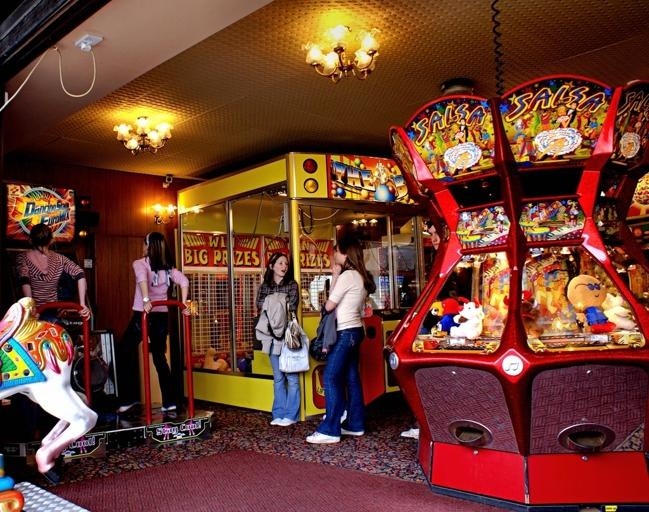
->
<box><xmin>305</xmin><ymin>231</ymin><xmax>375</xmax><ymax>442</ymax></box>
<box><xmin>259</xmin><ymin>252</ymin><xmax>302</xmax><ymax>426</ymax></box>
<box><xmin>399</xmin><ymin>217</ymin><xmax>442</xmax><ymax>440</ymax></box>
<box><xmin>14</xmin><ymin>223</ymin><xmax>91</xmax><ymax>320</ymax></box>
<box><xmin>109</xmin><ymin>231</ymin><xmax>189</xmax><ymax>413</ymax></box>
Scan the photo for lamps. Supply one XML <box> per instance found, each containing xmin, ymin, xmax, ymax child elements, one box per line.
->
<box><xmin>153</xmin><ymin>204</ymin><xmax>175</xmax><ymax>225</ymax></box>
<box><xmin>112</xmin><ymin>116</ymin><xmax>174</xmax><ymax>156</ymax></box>
<box><xmin>305</xmin><ymin>25</ymin><xmax>380</xmax><ymax>84</ymax></box>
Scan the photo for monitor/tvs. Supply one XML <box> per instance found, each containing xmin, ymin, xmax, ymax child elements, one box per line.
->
<box><xmin>379</xmin><ymin>274</ymin><xmax>403</xmax><ymax>302</ymax></box>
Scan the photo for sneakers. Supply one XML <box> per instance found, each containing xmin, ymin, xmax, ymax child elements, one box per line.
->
<box><xmin>340</xmin><ymin>427</ymin><xmax>364</xmax><ymax>436</ymax></box>
<box><xmin>400</xmin><ymin>428</ymin><xmax>419</xmax><ymax>439</ymax></box>
<box><xmin>119</xmin><ymin>401</ymin><xmax>137</xmax><ymax>413</ymax></box>
<box><xmin>270</xmin><ymin>418</ymin><xmax>297</xmax><ymax>427</ymax></box>
<box><xmin>306</xmin><ymin>432</ymin><xmax>341</xmax><ymax>443</ymax></box>
<box><xmin>161</xmin><ymin>405</ymin><xmax>177</xmax><ymax>412</ymax></box>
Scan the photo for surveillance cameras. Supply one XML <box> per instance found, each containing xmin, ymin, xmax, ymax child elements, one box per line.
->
<box><xmin>165</xmin><ymin>175</ymin><xmax>173</xmax><ymax>183</ymax></box>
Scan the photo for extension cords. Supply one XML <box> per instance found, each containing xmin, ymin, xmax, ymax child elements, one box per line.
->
<box><xmin>283</xmin><ymin>202</ymin><xmax>289</xmax><ymax>233</ymax></box>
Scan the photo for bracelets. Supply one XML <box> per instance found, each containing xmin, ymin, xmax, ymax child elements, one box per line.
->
<box><xmin>140</xmin><ymin>297</ymin><xmax>151</xmax><ymax>304</ymax></box>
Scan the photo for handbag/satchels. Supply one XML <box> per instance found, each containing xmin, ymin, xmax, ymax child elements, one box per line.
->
<box><xmin>285</xmin><ymin>304</ymin><xmax>302</xmax><ymax>350</ymax></box>
<box><xmin>309</xmin><ymin>337</ymin><xmax>334</xmax><ymax>362</ymax></box>
<box><xmin>279</xmin><ymin>303</ymin><xmax>310</xmax><ymax>373</ymax></box>
